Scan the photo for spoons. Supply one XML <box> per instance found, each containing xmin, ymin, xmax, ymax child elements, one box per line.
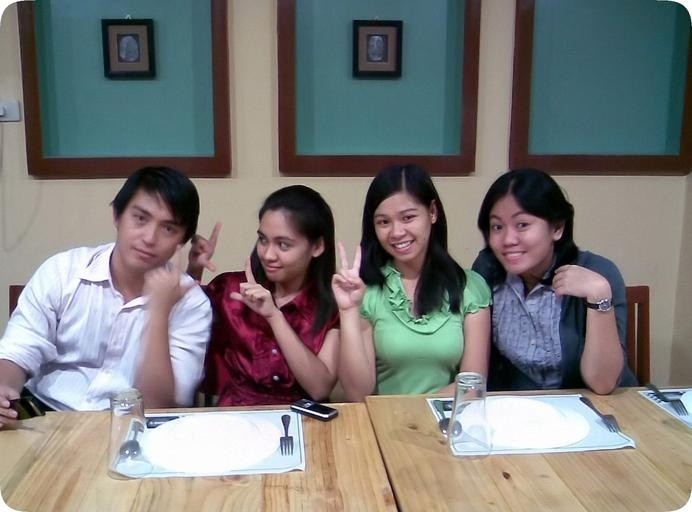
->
<box><xmin>433</xmin><ymin>399</ymin><xmax>467</xmax><ymax>438</ymax></box>
<box><xmin>120</xmin><ymin>421</ymin><xmax>145</xmax><ymax>460</ymax></box>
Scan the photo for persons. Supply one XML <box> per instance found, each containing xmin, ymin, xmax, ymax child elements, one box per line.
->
<box><xmin>331</xmin><ymin>164</ymin><xmax>494</xmax><ymax>402</ymax></box>
<box><xmin>471</xmin><ymin>169</ymin><xmax>639</xmax><ymax>393</ymax></box>
<box><xmin>0</xmin><ymin>165</ymin><xmax>214</xmax><ymax>432</ymax></box>
<box><xmin>185</xmin><ymin>185</ymin><xmax>342</xmax><ymax>408</ymax></box>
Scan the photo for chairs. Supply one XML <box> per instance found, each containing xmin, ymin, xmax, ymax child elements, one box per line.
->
<box><xmin>625</xmin><ymin>285</ymin><xmax>649</xmax><ymax>386</ymax></box>
<box><xmin>8</xmin><ymin>286</ymin><xmax>26</xmax><ymax>321</ymax></box>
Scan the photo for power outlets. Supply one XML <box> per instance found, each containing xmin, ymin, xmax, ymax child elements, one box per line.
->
<box><xmin>0</xmin><ymin>101</ymin><xmax>21</xmax><ymax>123</ymax></box>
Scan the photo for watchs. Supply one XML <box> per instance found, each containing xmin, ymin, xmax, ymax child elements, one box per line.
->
<box><xmin>586</xmin><ymin>298</ymin><xmax>614</xmax><ymax>312</ymax></box>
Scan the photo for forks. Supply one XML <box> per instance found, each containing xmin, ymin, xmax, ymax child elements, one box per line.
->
<box><xmin>644</xmin><ymin>382</ymin><xmax>688</xmax><ymax>416</ymax></box>
<box><xmin>279</xmin><ymin>414</ymin><xmax>295</xmax><ymax>455</ymax></box>
<box><xmin>579</xmin><ymin>397</ymin><xmax>621</xmax><ymax>433</ymax></box>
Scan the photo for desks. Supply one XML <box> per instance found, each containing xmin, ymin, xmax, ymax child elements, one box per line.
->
<box><xmin>0</xmin><ymin>402</ymin><xmax>399</xmax><ymax>512</ymax></box>
<box><xmin>366</xmin><ymin>385</ymin><xmax>692</xmax><ymax>512</ymax></box>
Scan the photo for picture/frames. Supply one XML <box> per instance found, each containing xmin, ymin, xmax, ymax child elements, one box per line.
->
<box><xmin>101</xmin><ymin>18</ymin><xmax>157</xmax><ymax>81</ymax></box>
<box><xmin>353</xmin><ymin>21</ymin><xmax>403</xmax><ymax>80</ymax></box>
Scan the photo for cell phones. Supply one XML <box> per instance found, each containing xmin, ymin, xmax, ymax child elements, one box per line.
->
<box><xmin>8</xmin><ymin>398</ymin><xmax>46</xmax><ymax>422</ymax></box>
<box><xmin>291</xmin><ymin>397</ymin><xmax>338</xmax><ymax>421</ymax></box>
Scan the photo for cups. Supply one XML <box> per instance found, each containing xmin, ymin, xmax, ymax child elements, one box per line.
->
<box><xmin>110</xmin><ymin>390</ymin><xmax>154</xmax><ymax>477</ymax></box>
<box><xmin>448</xmin><ymin>373</ymin><xmax>492</xmax><ymax>455</ymax></box>
